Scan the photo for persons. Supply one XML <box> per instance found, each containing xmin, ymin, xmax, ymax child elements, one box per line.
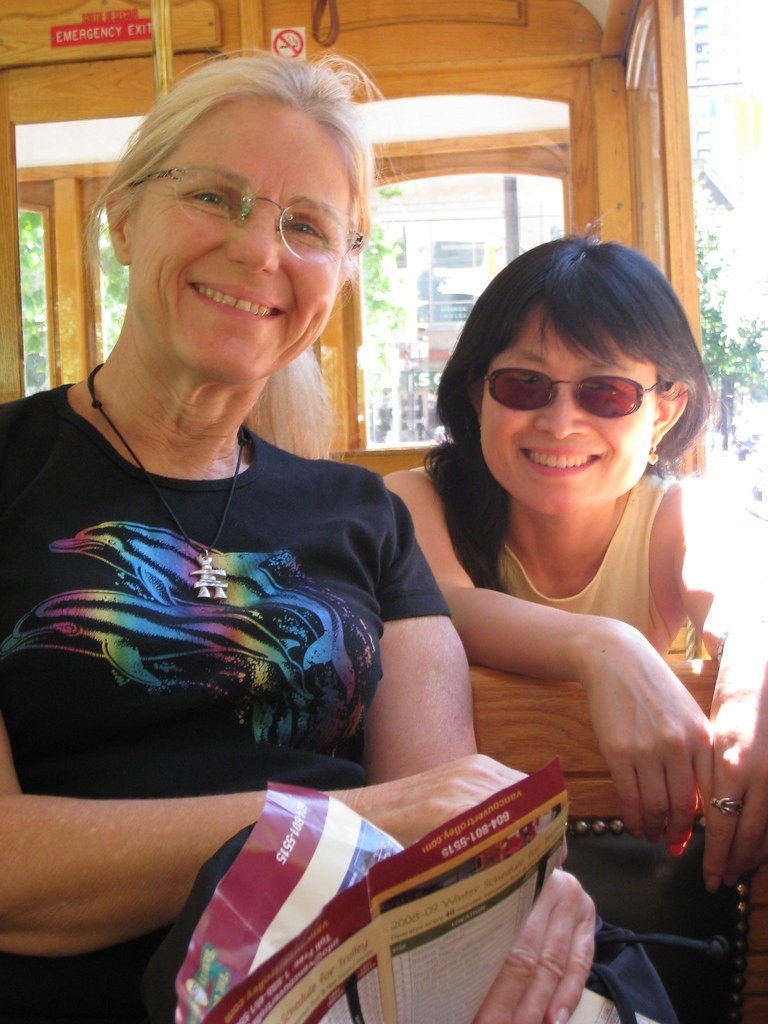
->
<box><xmin>380</xmin><ymin>237</ymin><xmax>768</xmax><ymax>1024</ymax></box>
<box><xmin>0</xmin><ymin>51</ymin><xmax>596</xmax><ymax>1024</ymax></box>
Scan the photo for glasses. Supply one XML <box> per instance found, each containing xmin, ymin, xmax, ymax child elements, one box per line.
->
<box><xmin>483</xmin><ymin>367</ymin><xmax>664</xmax><ymax>418</ymax></box>
<box><xmin>126</xmin><ymin>164</ymin><xmax>364</xmax><ymax>263</ymax></box>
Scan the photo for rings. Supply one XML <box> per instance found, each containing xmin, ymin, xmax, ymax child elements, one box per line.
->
<box><xmin>710</xmin><ymin>797</ymin><xmax>743</xmax><ymax>816</ymax></box>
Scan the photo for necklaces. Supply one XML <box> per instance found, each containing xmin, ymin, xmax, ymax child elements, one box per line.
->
<box><xmin>88</xmin><ymin>363</ymin><xmax>248</xmax><ymax>600</ymax></box>
